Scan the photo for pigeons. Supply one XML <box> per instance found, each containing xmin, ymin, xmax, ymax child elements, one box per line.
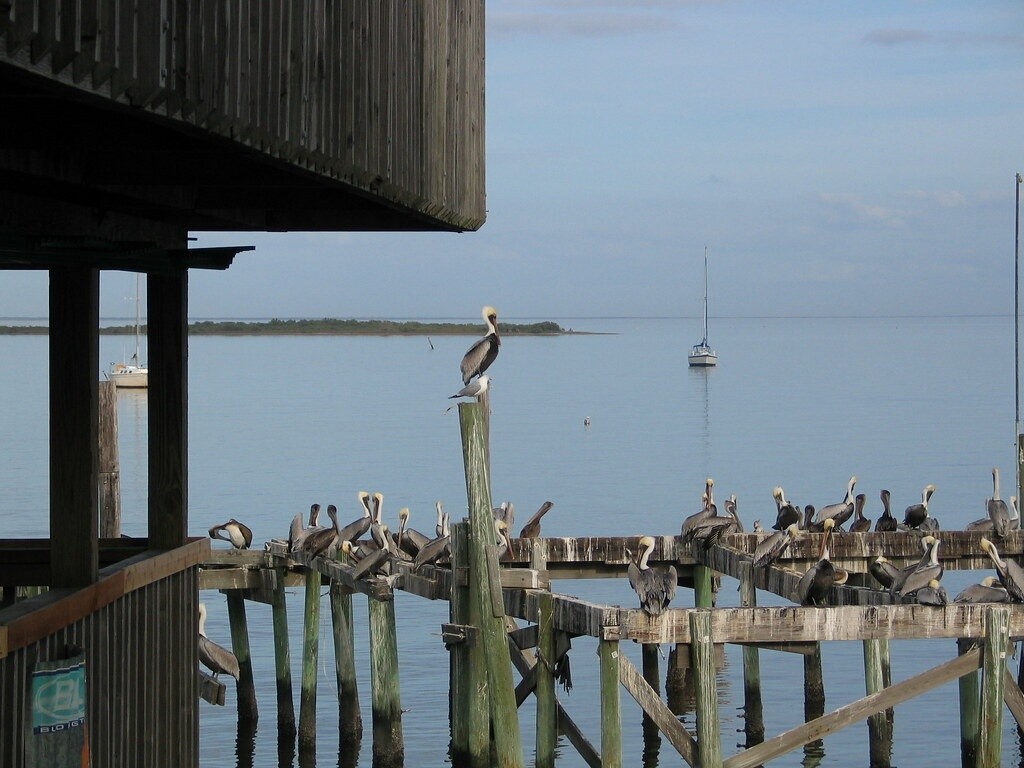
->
<box><xmin>448</xmin><ymin>373</ymin><xmax>492</xmax><ymax>403</ymax></box>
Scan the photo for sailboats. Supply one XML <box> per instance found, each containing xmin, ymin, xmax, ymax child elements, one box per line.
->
<box><xmin>102</xmin><ymin>271</ymin><xmax>148</xmax><ymax>388</ymax></box>
<box><xmin>686</xmin><ymin>244</ymin><xmax>718</xmax><ymax>366</ymax></box>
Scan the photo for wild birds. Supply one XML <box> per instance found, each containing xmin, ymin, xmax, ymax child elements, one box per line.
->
<box><xmin>198</xmin><ymin>602</ymin><xmax>241</xmax><ymax>682</ymax></box>
<box><xmin>680</xmin><ymin>467</ymin><xmax>1024</xmax><ymax>606</ymax></box>
<box><xmin>459</xmin><ymin>305</ymin><xmax>502</xmax><ymax>387</ymax></box>
<box><xmin>206</xmin><ymin>518</ymin><xmax>252</xmax><ymax>555</ymax></box>
<box><xmin>287</xmin><ymin>491</ymin><xmax>514</xmax><ymax>592</ymax></box>
<box><xmin>583</xmin><ymin>416</ymin><xmax>591</xmax><ymax>425</ymax></box>
<box><xmin>519</xmin><ymin>501</ymin><xmax>553</xmax><ymax>537</ymax></box>
<box><xmin>627</xmin><ymin>537</ymin><xmax>678</xmax><ymax>614</ymax></box>
